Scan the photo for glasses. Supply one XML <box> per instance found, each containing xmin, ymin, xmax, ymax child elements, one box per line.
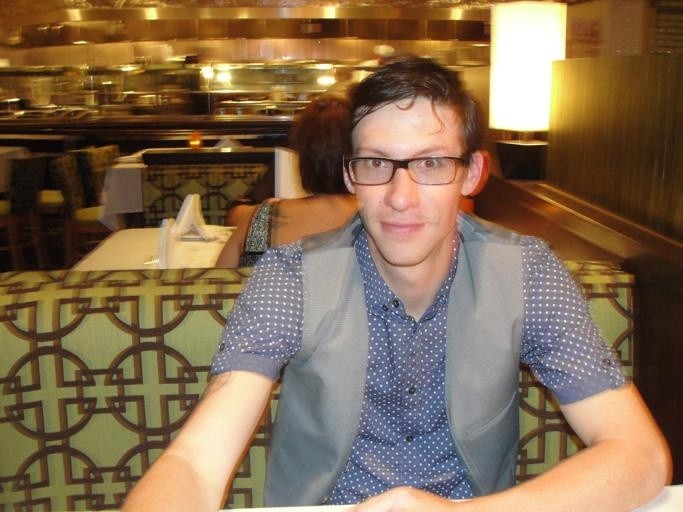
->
<box><xmin>340</xmin><ymin>156</ymin><xmax>468</xmax><ymax>187</ymax></box>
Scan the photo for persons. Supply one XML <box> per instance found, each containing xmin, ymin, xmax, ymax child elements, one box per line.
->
<box><xmin>115</xmin><ymin>55</ymin><xmax>672</xmax><ymax>512</ymax></box>
<box><xmin>208</xmin><ymin>87</ymin><xmax>359</xmax><ymax>270</ymax></box>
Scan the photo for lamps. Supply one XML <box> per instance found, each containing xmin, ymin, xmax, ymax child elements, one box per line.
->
<box><xmin>487</xmin><ymin>2</ymin><xmax>568</xmax><ymax>187</ymax></box>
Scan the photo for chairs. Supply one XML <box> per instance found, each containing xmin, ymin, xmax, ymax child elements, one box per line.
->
<box><xmin>1</xmin><ymin>145</ymin><xmax>121</xmax><ymax>270</ymax></box>
